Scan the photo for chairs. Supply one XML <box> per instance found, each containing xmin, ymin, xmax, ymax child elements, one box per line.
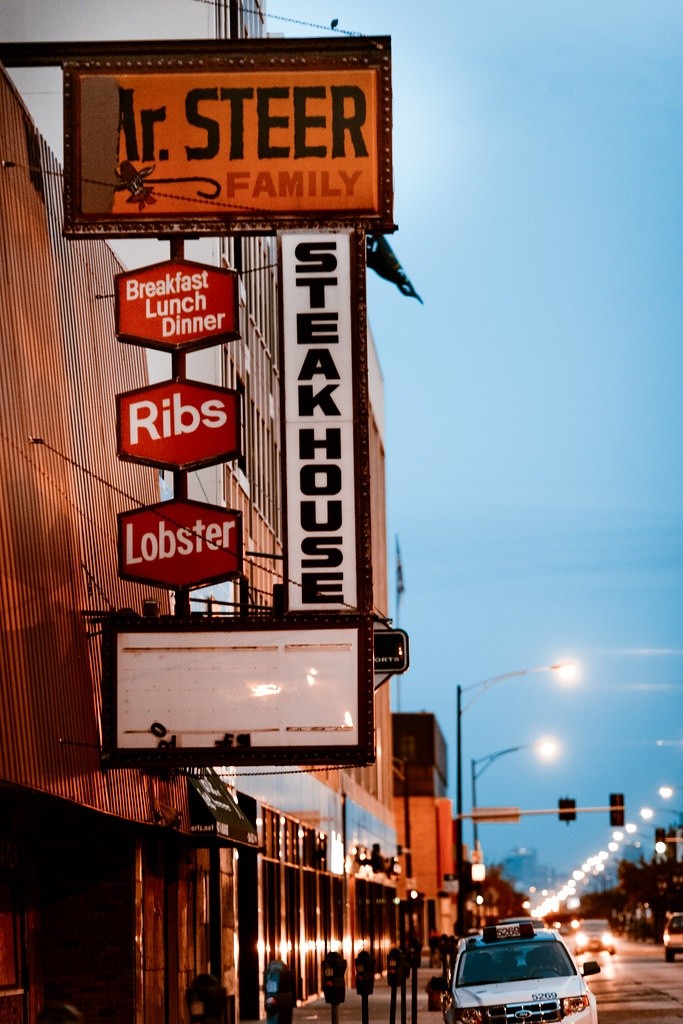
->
<box><xmin>525</xmin><ymin>948</ymin><xmax>561</xmax><ymax>974</ymax></box>
<box><xmin>464</xmin><ymin>953</ymin><xmax>503</xmax><ymax>983</ymax></box>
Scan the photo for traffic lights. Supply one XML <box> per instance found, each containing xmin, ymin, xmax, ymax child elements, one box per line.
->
<box><xmin>655</xmin><ymin>828</ymin><xmax>666</xmax><ymax>854</ymax></box>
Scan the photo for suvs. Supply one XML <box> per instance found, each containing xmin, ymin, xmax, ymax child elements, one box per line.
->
<box><xmin>572</xmin><ymin>919</ymin><xmax>615</xmax><ymax>956</ymax></box>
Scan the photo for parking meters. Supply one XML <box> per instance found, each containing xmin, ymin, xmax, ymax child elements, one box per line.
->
<box><xmin>320</xmin><ymin>952</ymin><xmax>347</xmax><ymax>1024</ymax></box>
<box><xmin>261</xmin><ymin>960</ymin><xmax>296</xmax><ymax>1024</ymax></box>
<box><xmin>387</xmin><ymin>948</ymin><xmax>410</xmax><ymax>1024</ymax></box>
<box><xmin>401</xmin><ymin>936</ymin><xmax>420</xmax><ymax>1024</ymax></box>
<box><xmin>185</xmin><ymin>974</ymin><xmax>228</xmax><ymax>1024</ymax></box>
<box><xmin>354</xmin><ymin>951</ymin><xmax>378</xmax><ymax>1024</ymax></box>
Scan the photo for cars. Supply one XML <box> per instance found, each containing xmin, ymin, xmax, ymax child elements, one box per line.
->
<box><xmin>497</xmin><ymin>917</ymin><xmax>549</xmax><ymax>929</ymax></box>
<box><xmin>442</xmin><ymin>923</ymin><xmax>605</xmax><ymax>1024</ymax></box>
<box><xmin>662</xmin><ymin>911</ymin><xmax>683</xmax><ymax>961</ymax></box>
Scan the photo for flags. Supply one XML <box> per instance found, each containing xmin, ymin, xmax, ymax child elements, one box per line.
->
<box><xmin>366</xmin><ymin>224</ymin><xmax>421</xmax><ymax>301</ymax></box>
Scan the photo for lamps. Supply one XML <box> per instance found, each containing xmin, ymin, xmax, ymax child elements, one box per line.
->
<box><xmin>381</xmin><ymin>857</ymin><xmax>402</xmax><ymax>878</ymax></box>
<box><xmin>359</xmin><ymin>843</ymin><xmax>384</xmax><ymax>873</ymax></box>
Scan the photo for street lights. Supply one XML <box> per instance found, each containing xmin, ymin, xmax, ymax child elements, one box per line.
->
<box><xmin>472</xmin><ymin>740</ymin><xmax>561</xmax><ymax>848</ymax></box>
<box><xmin>455</xmin><ymin>659</ymin><xmax>575</xmax><ymax>924</ymax></box>
